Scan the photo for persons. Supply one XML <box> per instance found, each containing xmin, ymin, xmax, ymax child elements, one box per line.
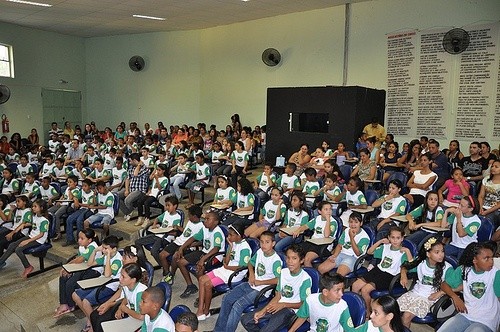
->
<box><xmin>174</xmin><ymin>313</ymin><xmax>198</xmax><ymax>332</ymax></box>
<box><xmin>114</xmin><ymin>263</ymin><xmax>149</xmax><ymax>332</ymax></box>
<box><xmin>203</xmin><ymin>231</ymin><xmax>284</xmax><ymax>332</ymax></box>
<box><xmin>72</xmin><ymin>235</ymin><xmax>124</xmax><ymax>332</ymax></box>
<box><xmin>87</xmin><ymin>245</ymin><xmax>148</xmax><ymax>332</ymax></box>
<box><xmin>239</xmin><ymin>242</ymin><xmax>314</xmax><ymax>332</ymax></box>
<box><xmin>438</xmin><ymin>240</ymin><xmax>500</xmax><ymax>332</ymax></box>
<box><xmin>0</xmin><ymin>113</ymin><xmax>499</xmax><ymax>308</ymax></box>
<box><xmin>288</xmin><ymin>272</ymin><xmax>355</xmax><ymax>332</ymax></box>
<box><xmin>394</xmin><ymin>236</ymin><xmax>455</xmax><ymax>332</ymax></box>
<box><xmin>350</xmin><ymin>225</ymin><xmax>415</xmax><ymax>322</ymax></box>
<box><xmin>350</xmin><ymin>294</ymin><xmax>413</xmax><ymax>332</ymax></box>
<box><xmin>194</xmin><ymin>222</ymin><xmax>253</xmax><ymax>322</ymax></box>
<box><xmin>52</xmin><ymin>227</ymin><xmax>102</xmax><ymax>319</ymax></box>
<box><xmin>137</xmin><ymin>286</ymin><xmax>176</xmax><ymax>332</ymax></box>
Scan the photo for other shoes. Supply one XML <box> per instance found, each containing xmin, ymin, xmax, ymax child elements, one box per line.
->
<box><xmin>126</xmin><ymin>212</ymin><xmax>133</xmax><ymax>221</ymax></box>
<box><xmin>62</xmin><ymin>238</ymin><xmax>76</xmax><ymax>247</ymax></box>
<box><xmin>141</xmin><ymin>218</ymin><xmax>151</xmax><ymax>228</ymax></box>
<box><xmin>52</xmin><ymin>233</ymin><xmax>62</xmax><ymax>242</ymax></box>
<box><xmin>163</xmin><ymin>268</ymin><xmax>170</xmax><ymax>276</ymax></box>
<box><xmin>135</xmin><ymin>216</ymin><xmax>146</xmax><ymax>226</ymax></box>
<box><xmin>74</xmin><ymin>243</ymin><xmax>79</xmax><ymax>249</ymax></box>
<box><xmin>207</xmin><ymin>313</ymin><xmax>211</xmax><ymax>319</ymax></box>
<box><xmin>124</xmin><ymin>207</ymin><xmax>135</xmax><ymax>219</ymax></box>
<box><xmin>184</xmin><ymin>202</ymin><xmax>195</xmax><ymax>210</ymax></box>
<box><xmin>196</xmin><ymin>314</ymin><xmax>206</xmax><ymax>321</ymax></box>
<box><xmin>179</xmin><ymin>284</ymin><xmax>198</xmax><ymax>298</ymax></box>
<box><xmin>159</xmin><ymin>272</ymin><xmax>173</xmax><ymax>285</ymax></box>
<box><xmin>194</xmin><ymin>297</ymin><xmax>199</xmax><ymax>307</ymax></box>
<box><xmin>81</xmin><ymin>325</ymin><xmax>90</xmax><ymax>332</ymax></box>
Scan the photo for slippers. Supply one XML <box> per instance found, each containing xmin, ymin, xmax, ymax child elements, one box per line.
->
<box><xmin>52</xmin><ymin>306</ymin><xmax>70</xmax><ymax>317</ymax></box>
<box><xmin>54</xmin><ymin>306</ymin><xmax>75</xmax><ymax>312</ymax></box>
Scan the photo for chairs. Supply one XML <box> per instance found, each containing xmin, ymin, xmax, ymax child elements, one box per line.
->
<box><xmin>0</xmin><ymin>121</ymin><xmax>500</xmax><ymax>331</ymax></box>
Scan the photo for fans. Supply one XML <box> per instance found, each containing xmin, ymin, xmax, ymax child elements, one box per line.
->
<box><xmin>262</xmin><ymin>48</ymin><xmax>281</xmax><ymax>67</ymax></box>
<box><xmin>443</xmin><ymin>28</ymin><xmax>470</xmax><ymax>55</ymax></box>
<box><xmin>129</xmin><ymin>56</ymin><xmax>145</xmax><ymax>72</ymax></box>
